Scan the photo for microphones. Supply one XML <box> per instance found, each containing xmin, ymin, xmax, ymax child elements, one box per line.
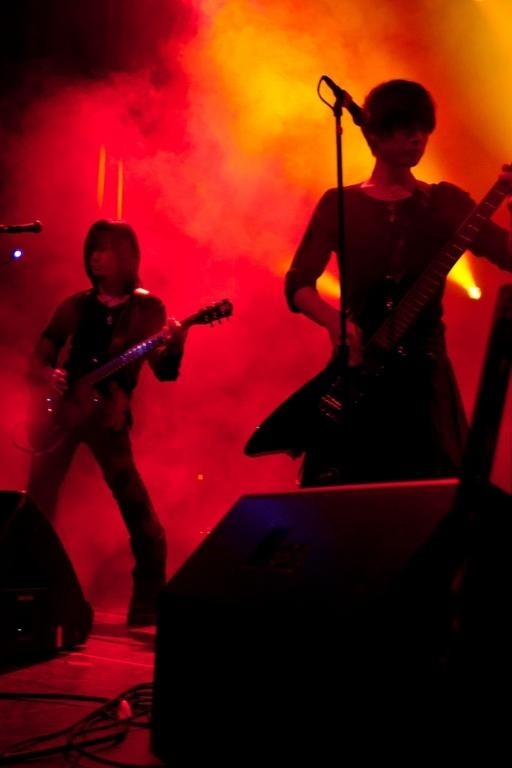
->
<box><xmin>320</xmin><ymin>71</ymin><xmax>369</xmax><ymax>130</ymax></box>
<box><xmin>1</xmin><ymin>219</ymin><xmax>46</xmax><ymax>235</ymax></box>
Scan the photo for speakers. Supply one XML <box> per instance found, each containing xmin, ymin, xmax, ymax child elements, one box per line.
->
<box><xmin>147</xmin><ymin>481</ymin><xmax>512</xmax><ymax>766</ymax></box>
<box><xmin>1</xmin><ymin>488</ymin><xmax>92</xmax><ymax>677</ymax></box>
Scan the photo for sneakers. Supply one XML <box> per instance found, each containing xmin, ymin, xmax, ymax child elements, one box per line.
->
<box><xmin>127</xmin><ymin>597</ymin><xmax>157</xmax><ymax>626</ymax></box>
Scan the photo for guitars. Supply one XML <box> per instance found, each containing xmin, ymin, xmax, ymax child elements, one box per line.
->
<box><xmin>28</xmin><ymin>299</ymin><xmax>232</xmax><ymax>452</ymax></box>
<box><xmin>242</xmin><ymin>161</ymin><xmax>512</xmax><ymax>460</ymax></box>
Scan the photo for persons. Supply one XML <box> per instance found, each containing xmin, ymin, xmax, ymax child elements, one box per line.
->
<box><xmin>285</xmin><ymin>79</ymin><xmax>512</xmax><ymax>489</ymax></box>
<box><xmin>26</xmin><ymin>218</ymin><xmax>187</xmax><ymax>627</ymax></box>
